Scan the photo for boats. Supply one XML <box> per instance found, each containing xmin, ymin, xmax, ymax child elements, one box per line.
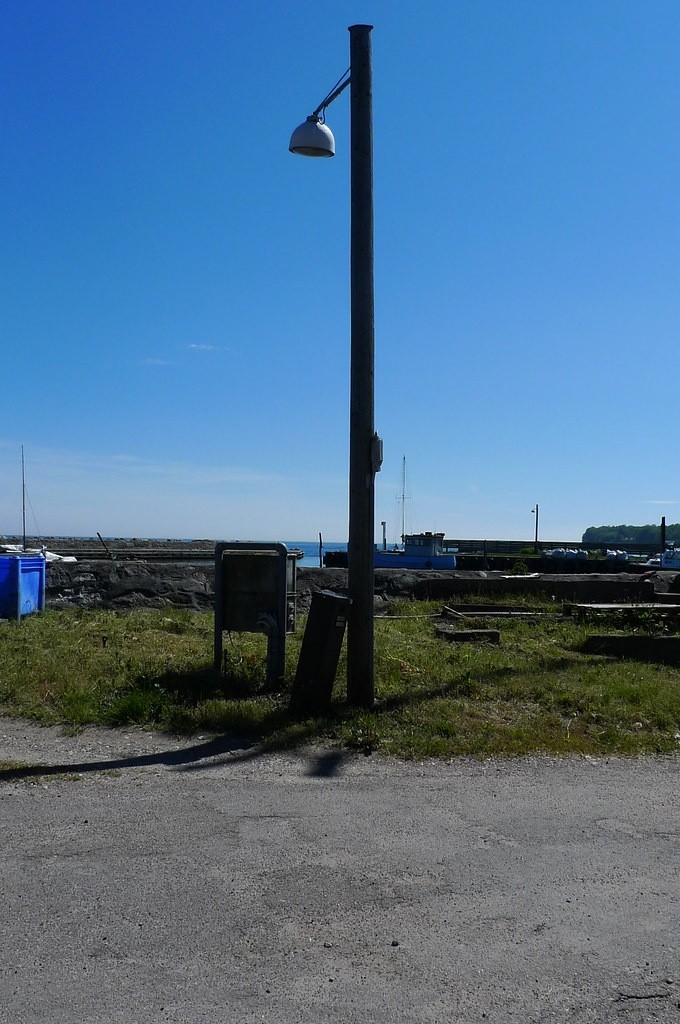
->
<box><xmin>288</xmin><ymin>548</ymin><xmax>305</xmax><ymax>559</ymax></box>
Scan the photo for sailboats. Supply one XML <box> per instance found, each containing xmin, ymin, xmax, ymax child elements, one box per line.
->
<box><xmin>0</xmin><ymin>444</ymin><xmax>79</xmax><ymax>564</ymax></box>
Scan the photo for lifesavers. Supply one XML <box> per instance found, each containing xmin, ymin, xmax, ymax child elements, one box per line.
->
<box><xmin>426</xmin><ymin>561</ymin><xmax>431</xmax><ymax>567</ymax></box>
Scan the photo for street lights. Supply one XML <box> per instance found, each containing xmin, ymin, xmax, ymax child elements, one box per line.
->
<box><xmin>288</xmin><ymin>22</ymin><xmax>383</xmax><ymax>711</ymax></box>
<box><xmin>531</xmin><ymin>503</ymin><xmax>539</xmax><ymax>554</ymax></box>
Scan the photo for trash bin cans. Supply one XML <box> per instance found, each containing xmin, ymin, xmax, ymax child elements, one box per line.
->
<box><xmin>0</xmin><ymin>553</ymin><xmax>46</xmax><ymax>626</ymax></box>
